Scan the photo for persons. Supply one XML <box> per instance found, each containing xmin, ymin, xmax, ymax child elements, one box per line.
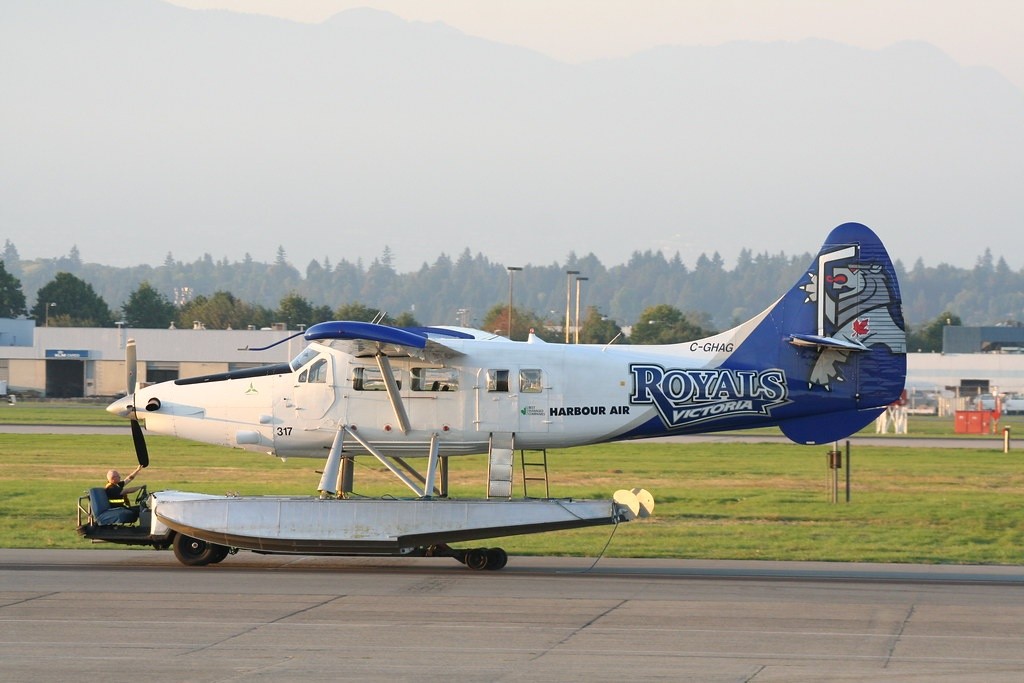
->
<box><xmin>442</xmin><ymin>385</ymin><xmax>450</xmax><ymax>392</ymax></box>
<box><xmin>431</xmin><ymin>381</ymin><xmax>441</xmax><ymax>391</ymax></box>
<box><xmin>105</xmin><ymin>465</ymin><xmax>146</xmax><ymax>528</ymax></box>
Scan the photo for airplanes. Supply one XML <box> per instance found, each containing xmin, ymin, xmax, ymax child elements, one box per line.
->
<box><xmin>105</xmin><ymin>220</ymin><xmax>908</xmax><ymax>571</ymax></box>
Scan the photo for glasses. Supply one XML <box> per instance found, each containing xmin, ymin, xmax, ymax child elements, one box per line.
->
<box><xmin>113</xmin><ymin>475</ymin><xmax>119</xmax><ymax>477</ymax></box>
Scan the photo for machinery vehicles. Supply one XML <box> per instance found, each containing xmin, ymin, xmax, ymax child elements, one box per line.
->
<box><xmin>76</xmin><ymin>483</ymin><xmax>232</xmax><ymax>546</ymax></box>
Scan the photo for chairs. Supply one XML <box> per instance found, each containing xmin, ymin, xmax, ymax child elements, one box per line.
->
<box><xmin>395</xmin><ymin>380</ymin><xmax>402</xmax><ymax>391</ymax></box>
<box><xmin>90</xmin><ymin>488</ymin><xmax>137</xmax><ymax>524</ymax></box>
<box><xmin>442</xmin><ymin>384</ymin><xmax>449</xmax><ymax>391</ymax></box>
<box><xmin>431</xmin><ymin>381</ymin><xmax>440</xmax><ymax>390</ymax></box>
